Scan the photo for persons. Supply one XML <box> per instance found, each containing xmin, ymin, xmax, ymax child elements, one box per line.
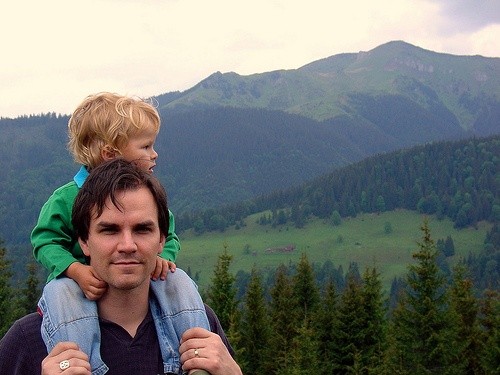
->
<box><xmin>0</xmin><ymin>157</ymin><xmax>243</xmax><ymax>375</ymax></box>
<box><xmin>30</xmin><ymin>92</ymin><xmax>211</xmax><ymax>375</ymax></box>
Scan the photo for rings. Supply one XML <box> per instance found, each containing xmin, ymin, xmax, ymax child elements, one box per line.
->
<box><xmin>194</xmin><ymin>348</ymin><xmax>200</xmax><ymax>358</ymax></box>
<box><xmin>59</xmin><ymin>360</ymin><xmax>70</xmax><ymax>370</ymax></box>
